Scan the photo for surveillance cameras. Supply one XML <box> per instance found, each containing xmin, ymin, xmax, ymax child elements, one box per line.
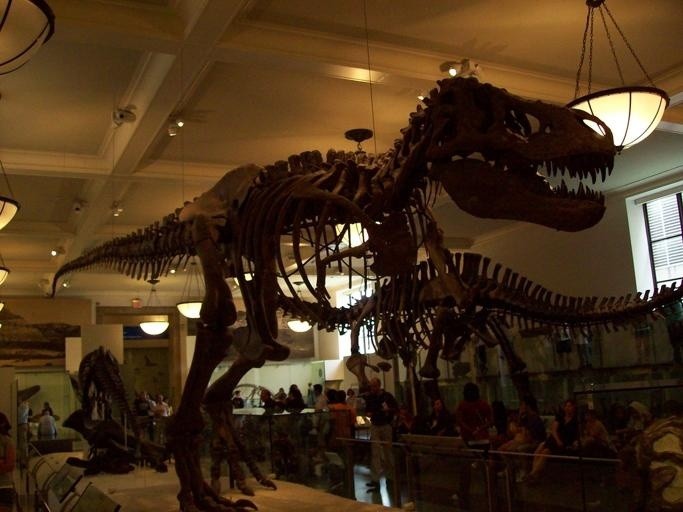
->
<box><xmin>462</xmin><ymin>61</ymin><xmax>481</xmax><ymax>76</ymax></box>
<box><xmin>72</xmin><ymin>200</ymin><xmax>82</xmax><ymax>213</ymax></box>
<box><xmin>112</xmin><ymin>107</ymin><xmax>126</xmax><ymax>122</ymax></box>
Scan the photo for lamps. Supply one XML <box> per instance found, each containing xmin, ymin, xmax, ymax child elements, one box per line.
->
<box><xmin>563</xmin><ymin>1</ymin><xmax>670</xmax><ymax>155</ymax></box>
<box><xmin>168</xmin><ymin>115</ymin><xmax>185</xmax><ymax>137</ymax></box>
<box><xmin>51</xmin><ymin>248</ymin><xmax>65</xmax><ymax>257</ymax></box>
<box><xmin>333</xmin><ymin>129</ymin><xmax>382</xmax><ymax>249</ymax></box>
<box><xmin>1</xmin><ymin>0</ymin><xmax>56</xmax><ymax>76</ymax></box>
<box><xmin>440</xmin><ymin>61</ymin><xmax>462</xmax><ymax>78</ymax></box>
<box><xmin>287</xmin><ymin>282</ymin><xmax>313</xmax><ymax>333</ymax></box>
<box><xmin>139</xmin><ymin>261</ymin><xmax>170</xmax><ymax>336</ymax></box>
<box><xmin>0</xmin><ymin>254</ymin><xmax>9</xmax><ymax>285</ymax></box>
<box><xmin>0</xmin><ymin>159</ymin><xmax>21</xmax><ymax>230</ymax></box>
<box><xmin>176</xmin><ymin>256</ymin><xmax>206</xmax><ymax>319</ymax></box>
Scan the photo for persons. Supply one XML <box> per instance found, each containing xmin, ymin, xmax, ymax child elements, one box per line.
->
<box><xmin>0</xmin><ymin>411</ymin><xmax>15</xmax><ymax>477</ymax></box>
<box><xmin>234</xmin><ymin>382</ymin><xmax>683</xmax><ymax>512</ymax></box>
<box><xmin>39</xmin><ymin>410</ymin><xmax>55</xmax><ymax>440</ymax></box>
<box><xmin>132</xmin><ymin>388</ymin><xmax>171</xmax><ymax>445</ymax></box>
<box><xmin>35</xmin><ymin>401</ymin><xmax>53</xmax><ymax>418</ymax></box>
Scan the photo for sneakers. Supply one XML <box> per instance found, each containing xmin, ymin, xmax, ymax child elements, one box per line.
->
<box><xmin>364</xmin><ymin>478</ymin><xmax>381</xmax><ymax>490</ymax></box>
<box><xmin>497</xmin><ymin>468</ymin><xmax>541</xmax><ymax>484</ymax></box>
